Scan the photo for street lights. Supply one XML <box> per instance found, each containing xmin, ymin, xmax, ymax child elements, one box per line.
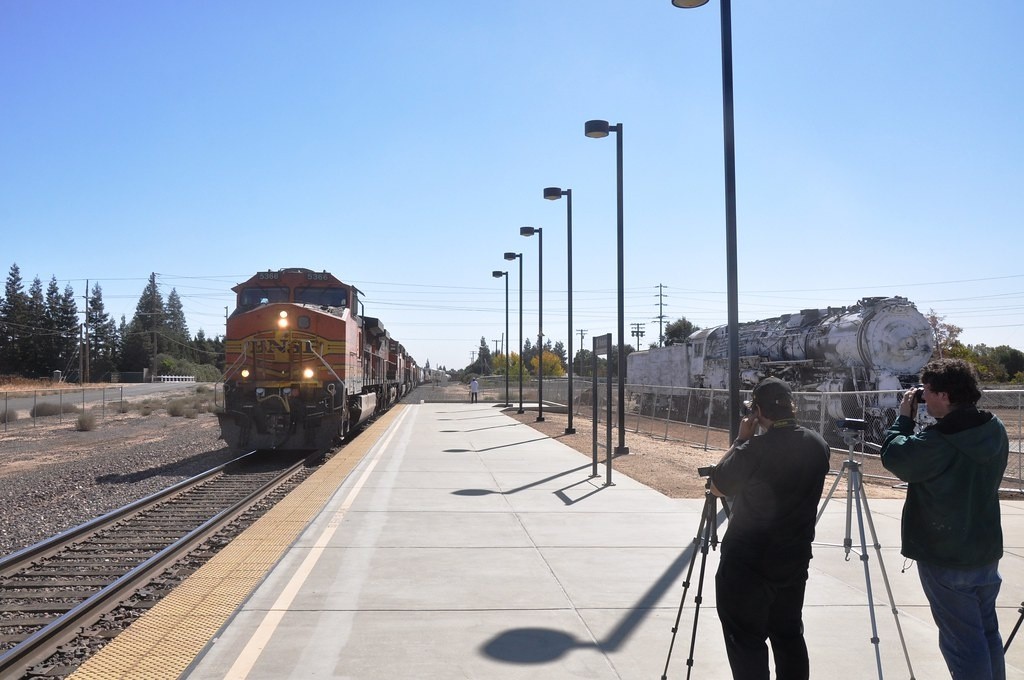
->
<box><xmin>584</xmin><ymin>119</ymin><xmax>630</xmax><ymax>455</ymax></box>
<box><xmin>520</xmin><ymin>226</ymin><xmax>545</xmax><ymax>423</ymax></box>
<box><xmin>542</xmin><ymin>187</ymin><xmax>576</xmax><ymax>436</ymax></box>
<box><xmin>672</xmin><ymin>1</ymin><xmax>740</xmax><ymax>450</ymax></box>
<box><xmin>492</xmin><ymin>270</ymin><xmax>514</xmax><ymax>407</ymax></box>
<box><xmin>503</xmin><ymin>252</ymin><xmax>524</xmax><ymax>414</ymax></box>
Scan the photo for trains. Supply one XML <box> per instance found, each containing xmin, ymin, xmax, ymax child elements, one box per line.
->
<box><xmin>213</xmin><ymin>269</ymin><xmax>451</xmax><ymax>451</ymax></box>
<box><xmin>626</xmin><ymin>295</ymin><xmax>940</xmax><ymax>452</ymax></box>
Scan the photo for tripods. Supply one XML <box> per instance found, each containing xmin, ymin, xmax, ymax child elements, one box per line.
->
<box><xmin>663</xmin><ymin>464</ymin><xmax>731</xmax><ymax>680</ymax></box>
<box><xmin>814</xmin><ymin>430</ymin><xmax>916</xmax><ymax>680</ymax></box>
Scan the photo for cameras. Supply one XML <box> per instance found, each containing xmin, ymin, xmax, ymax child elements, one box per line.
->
<box><xmin>914</xmin><ymin>384</ymin><xmax>926</xmax><ymax>403</ymax></box>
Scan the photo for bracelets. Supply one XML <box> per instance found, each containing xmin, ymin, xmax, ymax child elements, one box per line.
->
<box><xmin>733</xmin><ymin>437</ymin><xmax>745</xmax><ymax>445</ymax></box>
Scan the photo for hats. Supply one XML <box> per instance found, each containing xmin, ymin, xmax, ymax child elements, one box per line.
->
<box><xmin>753</xmin><ymin>376</ymin><xmax>791</xmax><ymax>406</ymax></box>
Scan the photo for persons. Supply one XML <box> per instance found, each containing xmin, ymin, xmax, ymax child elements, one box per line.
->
<box><xmin>881</xmin><ymin>359</ymin><xmax>1009</xmax><ymax>680</ymax></box>
<box><xmin>469</xmin><ymin>378</ymin><xmax>479</xmax><ymax>402</ymax></box>
<box><xmin>709</xmin><ymin>377</ymin><xmax>831</xmax><ymax>679</ymax></box>
